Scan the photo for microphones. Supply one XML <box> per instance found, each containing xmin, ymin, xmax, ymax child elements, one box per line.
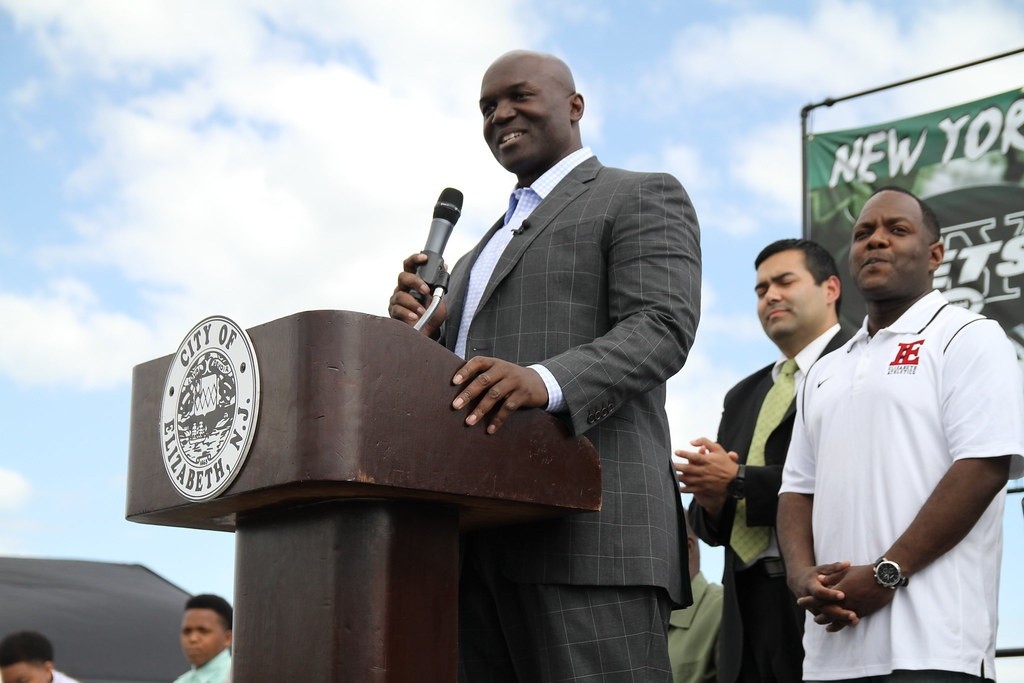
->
<box><xmin>408</xmin><ymin>188</ymin><xmax>464</xmax><ymax>306</ymax></box>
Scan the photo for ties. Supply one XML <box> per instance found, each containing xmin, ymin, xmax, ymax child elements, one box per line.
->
<box><xmin>729</xmin><ymin>359</ymin><xmax>799</xmax><ymax>564</ymax></box>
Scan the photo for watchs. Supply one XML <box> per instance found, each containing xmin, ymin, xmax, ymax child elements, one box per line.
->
<box><xmin>873</xmin><ymin>556</ymin><xmax>909</xmax><ymax>589</ymax></box>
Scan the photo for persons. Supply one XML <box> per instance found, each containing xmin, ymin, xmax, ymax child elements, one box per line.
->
<box><xmin>0</xmin><ymin>631</ymin><xmax>78</xmax><ymax>683</ymax></box>
<box><xmin>776</xmin><ymin>187</ymin><xmax>1024</xmax><ymax>683</ymax></box>
<box><xmin>390</xmin><ymin>52</ymin><xmax>702</xmax><ymax>683</ymax></box>
<box><xmin>666</xmin><ymin>239</ymin><xmax>853</xmax><ymax>683</ymax></box>
<box><xmin>171</xmin><ymin>594</ymin><xmax>233</xmax><ymax>683</ymax></box>
